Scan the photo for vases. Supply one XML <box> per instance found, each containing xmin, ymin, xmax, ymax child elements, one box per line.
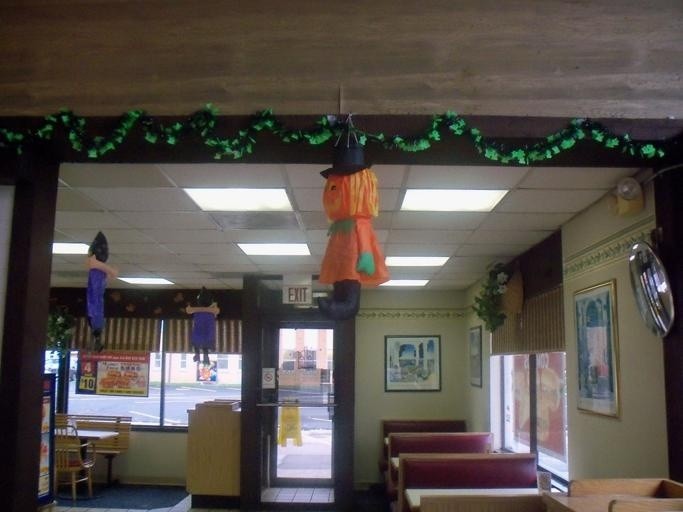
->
<box><xmin>502</xmin><ymin>267</ymin><xmax>523</xmax><ymax>317</ymax></box>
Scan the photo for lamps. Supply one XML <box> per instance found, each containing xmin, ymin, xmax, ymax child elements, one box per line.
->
<box><xmin>605</xmin><ymin>177</ymin><xmax>647</xmax><ymax>218</ymax></box>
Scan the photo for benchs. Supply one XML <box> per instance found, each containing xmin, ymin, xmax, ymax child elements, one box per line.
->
<box><xmin>375</xmin><ymin>416</ymin><xmax>569</xmax><ymax>512</ymax></box>
<box><xmin>54</xmin><ymin>412</ymin><xmax>131</xmax><ymax>505</ymax></box>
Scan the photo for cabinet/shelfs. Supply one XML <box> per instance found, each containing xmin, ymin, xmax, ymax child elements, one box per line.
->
<box><xmin>542</xmin><ymin>473</ymin><xmax>681</xmax><ymax>512</ymax></box>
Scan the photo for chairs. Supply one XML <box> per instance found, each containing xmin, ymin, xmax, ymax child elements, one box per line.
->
<box><xmin>55</xmin><ymin>424</ymin><xmax>97</xmax><ymax>501</ymax></box>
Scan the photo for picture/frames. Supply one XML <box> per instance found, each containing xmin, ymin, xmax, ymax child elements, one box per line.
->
<box><xmin>384</xmin><ymin>335</ymin><xmax>441</xmax><ymax>392</ymax></box>
<box><xmin>571</xmin><ymin>278</ymin><xmax>621</xmax><ymax>419</ymax></box>
<box><xmin>467</xmin><ymin>325</ymin><xmax>482</xmax><ymax>388</ymax></box>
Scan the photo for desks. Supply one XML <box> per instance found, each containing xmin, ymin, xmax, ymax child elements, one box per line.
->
<box><xmin>55</xmin><ymin>428</ymin><xmax>120</xmax><ymax>499</ymax></box>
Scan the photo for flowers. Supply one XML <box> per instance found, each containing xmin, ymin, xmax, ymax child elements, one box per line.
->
<box><xmin>470</xmin><ymin>260</ymin><xmax>517</xmax><ymax>331</ymax></box>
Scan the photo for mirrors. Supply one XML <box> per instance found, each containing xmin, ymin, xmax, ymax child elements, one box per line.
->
<box><xmin>627</xmin><ymin>241</ymin><xmax>675</xmax><ymax>338</ymax></box>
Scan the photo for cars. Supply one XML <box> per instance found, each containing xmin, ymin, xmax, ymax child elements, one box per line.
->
<box><xmin>46</xmin><ymin>358</ymin><xmax>77</xmax><ymax>383</ymax></box>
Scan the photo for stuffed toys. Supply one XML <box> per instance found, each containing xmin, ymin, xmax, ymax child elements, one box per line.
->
<box><xmin>87</xmin><ymin>232</ymin><xmax>117</xmax><ymax>352</ymax></box>
<box><xmin>187</xmin><ymin>286</ymin><xmax>221</xmax><ymax>363</ymax></box>
<box><xmin>318</xmin><ymin>143</ymin><xmax>391</xmax><ymax>320</ymax></box>
